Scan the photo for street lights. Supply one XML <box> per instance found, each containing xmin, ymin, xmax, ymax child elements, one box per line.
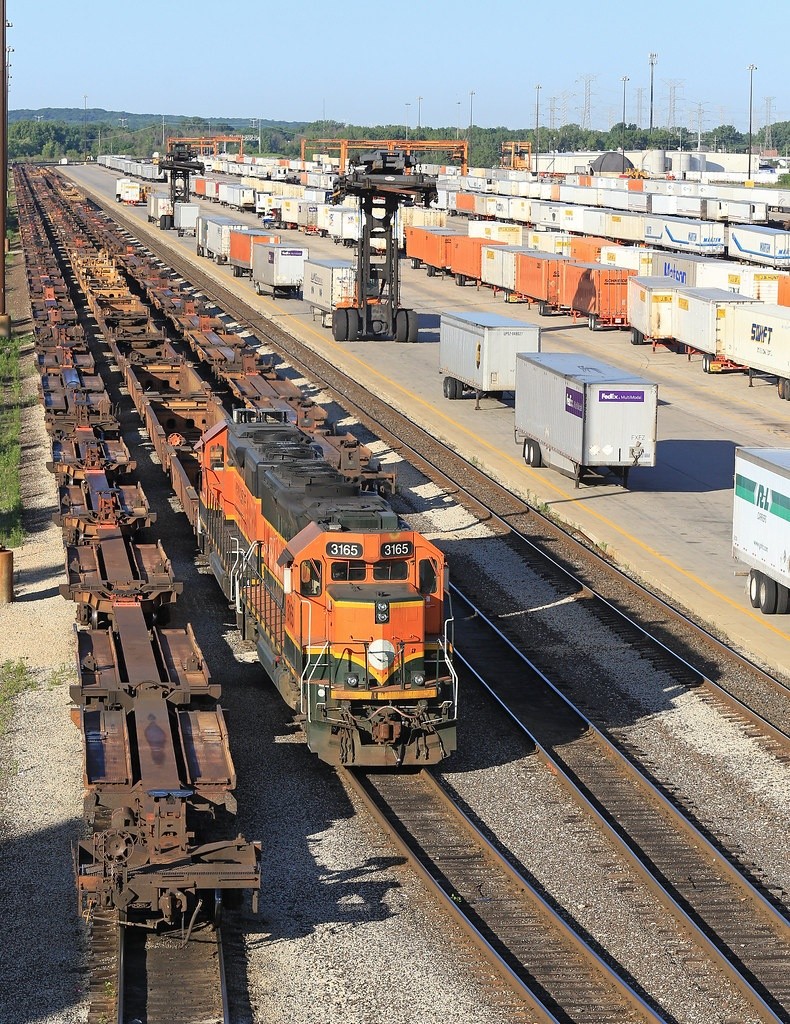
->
<box><xmin>405</xmin><ymin>103</ymin><xmax>411</xmax><ymax>140</ymax></box>
<box><xmin>417</xmin><ymin>95</ymin><xmax>423</xmax><ymax>165</ymax></box>
<box><xmin>647</xmin><ymin>52</ymin><xmax>659</xmax><ymax>134</ymax></box>
<box><xmin>456</xmin><ymin>102</ymin><xmax>461</xmax><ymax>140</ymax></box>
<box><xmin>468</xmin><ymin>90</ymin><xmax>476</xmax><ymax>168</ymax></box>
<box><xmin>619</xmin><ymin>76</ymin><xmax>630</xmax><ymax>174</ymax></box>
<box><xmin>6</xmin><ymin>46</ymin><xmax>15</xmax><ymax>207</ymax></box>
<box><xmin>534</xmin><ymin>85</ymin><xmax>542</xmax><ymax>173</ymax></box>
<box><xmin>81</xmin><ymin>95</ymin><xmax>88</xmax><ymax>161</ymax></box>
<box><xmin>746</xmin><ymin>62</ymin><xmax>758</xmax><ymax>180</ymax></box>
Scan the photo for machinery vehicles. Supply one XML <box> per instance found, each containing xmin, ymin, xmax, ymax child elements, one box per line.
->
<box><xmin>329</xmin><ymin>149</ymin><xmax>440</xmax><ymax>344</ymax></box>
<box><xmin>158</xmin><ymin>141</ymin><xmax>207</xmax><ymax>231</ymax></box>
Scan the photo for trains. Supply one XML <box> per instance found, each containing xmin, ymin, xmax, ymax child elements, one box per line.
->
<box><xmin>21</xmin><ymin>164</ymin><xmax>461</xmax><ymax>768</ymax></box>
